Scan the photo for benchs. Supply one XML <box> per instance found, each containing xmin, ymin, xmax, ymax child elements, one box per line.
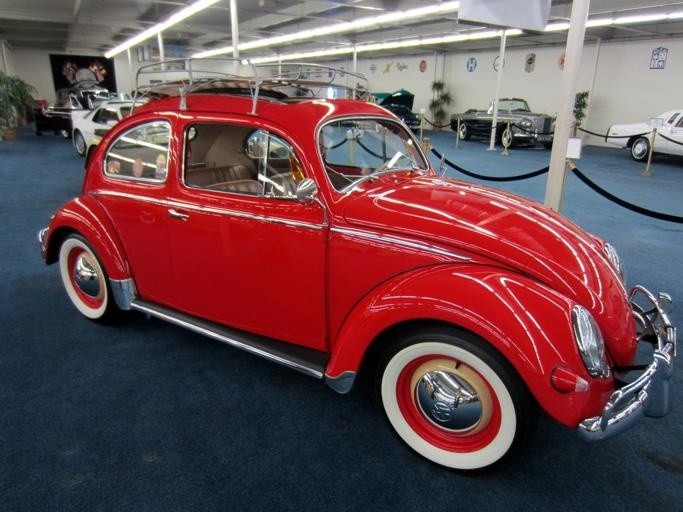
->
<box><xmin>184</xmin><ymin>165</ymin><xmax>252</xmax><ymax>188</ymax></box>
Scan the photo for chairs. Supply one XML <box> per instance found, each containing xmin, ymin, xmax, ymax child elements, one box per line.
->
<box><xmin>265</xmin><ymin>173</ymin><xmax>292</xmax><ymax>198</ymax></box>
<box><xmin>202</xmin><ymin>180</ymin><xmax>265</xmax><ymax>194</ymax></box>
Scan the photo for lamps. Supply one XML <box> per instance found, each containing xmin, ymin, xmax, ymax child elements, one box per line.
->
<box><xmin>102</xmin><ymin>1</ymin><xmax>683</xmax><ymax>67</ymax></box>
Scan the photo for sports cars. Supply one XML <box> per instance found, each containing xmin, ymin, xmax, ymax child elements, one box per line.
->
<box><xmin>448</xmin><ymin>97</ymin><xmax>556</xmax><ymax>148</ymax></box>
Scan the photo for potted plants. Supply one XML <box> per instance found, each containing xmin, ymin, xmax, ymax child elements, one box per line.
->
<box><xmin>0</xmin><ymin>70</ymin><xmax>42</xmax><ymax>142</ymax></box>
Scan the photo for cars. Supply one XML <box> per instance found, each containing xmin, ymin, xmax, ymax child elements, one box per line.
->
<box><xmin>378</xmin><ymin>103</ymin><xmax>421</xmax><ymax>137</ymax></box>
<box><xmin>603</xmin><ymin>109</ymin><xmax>683</xmax><ymax>161</ymax></box>
<box><xmin>36</xmin><ymin>55</ymin><xmax>677</xmax><ymax>475</ymax></box>
<box><xmin>34</xmin><ymin>87</ymin><xmax>121</xmax><ymax>139</ymax></box>
<box><xmin>69</xmin><ymin>99</ymin><xmax>171</xmax><ymax>157</ymax></box>
